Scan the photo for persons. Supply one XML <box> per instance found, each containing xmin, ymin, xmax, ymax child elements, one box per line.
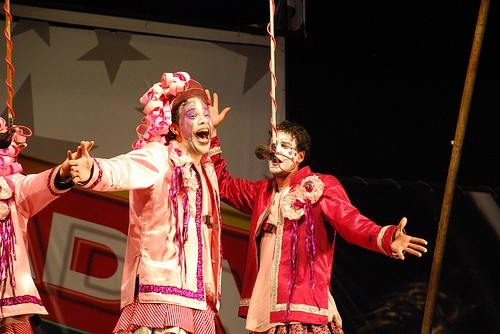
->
<box><xmin>67</xmin><ymin>70</ymin><xmax>222</xmax><ymax>334</ymax></box>
<box><xmin>201</xmin><ymin>88</ymin><xmax>429</xmax><ymax>334</ymax></box>
<box><xmin>0</xmin><ymin>115</ymin><xmax>97</xmax><ymax>334</ymax></box>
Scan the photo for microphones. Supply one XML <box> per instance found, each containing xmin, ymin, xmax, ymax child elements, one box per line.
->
<box><xmin>0</xmin><ymin>128</ymin><xmax>15</xmax><ymax>149</ymax></box>
<box><xmin>253</xmin><ymin>144</ymin><xmax>276</xmax><ymax>160</ymax></box>
